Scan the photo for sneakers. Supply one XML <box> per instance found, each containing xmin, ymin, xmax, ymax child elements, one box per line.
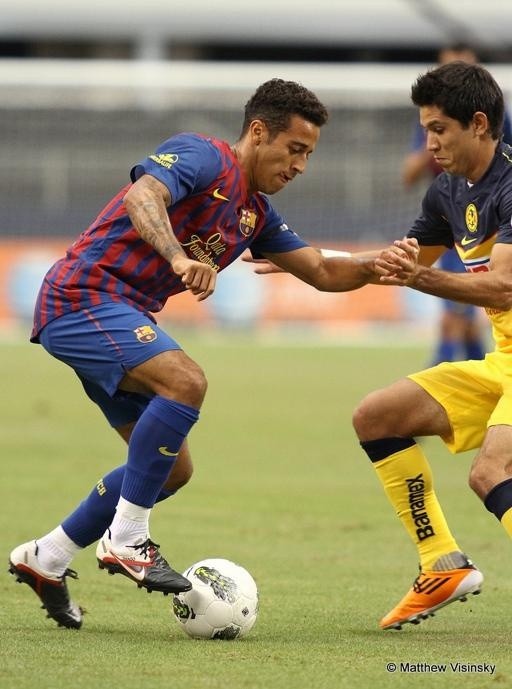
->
<box><xmin>95</xmin><ymin>532</ymin><xmax>193</xmax><ymax>596</ymax></box>
<box><xmin>376</xmin><ymin>552</ymin><xmax>485</xmax><ymax>631</ymax></box>
<box><xmin>7</xmin><ymin>538</ymin><xmax>84</xmax><ymax>630</ymax></box>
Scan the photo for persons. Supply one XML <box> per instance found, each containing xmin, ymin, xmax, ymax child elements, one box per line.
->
<box><xmin>236</xmin><ymin>57</ymin><xmax>512</xmax><ymax>631</ymax></box>
<box><xmin>8</xmin><ymin>78</ymin><xmax>420</xmax><ymax>630</ymax></box>
<box><xmin>399</xmin><ymin>45</ymin><xmax>510</xmax><ymax>365</ymax></box>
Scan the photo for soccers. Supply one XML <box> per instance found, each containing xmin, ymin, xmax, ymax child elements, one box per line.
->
<box><xmin>172</xmin><ymin>558</ymin><xmax>259</xmax><ymax>640</ymax></box>
